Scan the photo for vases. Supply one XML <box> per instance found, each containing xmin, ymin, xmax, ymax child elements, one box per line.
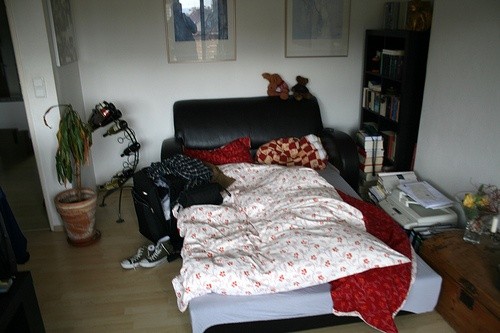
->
<box><xmin>456</xmin><ymin>191</ymin><xmax>500</xmax><ymax>244</ymax></box>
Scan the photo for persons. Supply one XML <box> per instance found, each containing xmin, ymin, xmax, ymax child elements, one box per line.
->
<box><xmin>173</xmin><ymin>2</ymin><xmax>197</xmax><ymax>42</ymax></box>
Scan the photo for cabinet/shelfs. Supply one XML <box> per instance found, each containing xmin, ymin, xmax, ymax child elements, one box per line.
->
<box><xmin>361</xmin><ymin>28</ymin><xmax>431</xmax><ymax>171</ymax></box>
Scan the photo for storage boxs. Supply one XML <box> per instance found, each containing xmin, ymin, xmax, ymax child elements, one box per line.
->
<box><xmin>421</xmin><ymin>230</ymin><xmax>500</xmax><ymax>333</ymax></box>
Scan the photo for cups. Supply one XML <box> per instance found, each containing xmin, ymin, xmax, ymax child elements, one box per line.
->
<box><xmin>462</xmin><ymin>220</ymin><xmax>483</xmax><ymax>246</ymax></box>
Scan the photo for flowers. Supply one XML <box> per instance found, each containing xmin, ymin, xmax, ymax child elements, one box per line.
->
<box><xmin>463</xmin><ymin>181</ymin><xmax>500</xmax><ymax>212</ymax></box>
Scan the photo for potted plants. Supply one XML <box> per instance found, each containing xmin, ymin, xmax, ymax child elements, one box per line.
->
<box><xmin>43</xmin><ymin>103</ymin><xmax>102</xmax><ymax>247</ymax></box>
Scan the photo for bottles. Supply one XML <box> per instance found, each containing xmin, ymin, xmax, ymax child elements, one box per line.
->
<box><xmin>120</xmin><ymin>143</ymin><xmax>140</xmax><ymax>157</ymax></box>
<box><xmin>87</xmin><ymin>101</ymin><xmax>128</xmax><ymax>137</ymax></box>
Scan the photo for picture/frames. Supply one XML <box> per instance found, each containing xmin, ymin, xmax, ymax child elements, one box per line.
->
<box><xmin>163</xmin><ymin>0</ymin><xmax>238</xmax><ymax>64</ymax></box>
<box><xmin>285</xmin><ymin>0</ymin><xmax>350</xmax><ymax>57</ymax></box>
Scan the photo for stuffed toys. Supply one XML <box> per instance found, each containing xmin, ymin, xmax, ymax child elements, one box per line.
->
<box><xmin>262</xmin><ymin>73</ymin><xmax>289</xmax><ymax>99</ymax></box>
<box><xmin>291</xmin><ymin>76</ymin><xmax>313</xmax><ymax>101</ymax></box>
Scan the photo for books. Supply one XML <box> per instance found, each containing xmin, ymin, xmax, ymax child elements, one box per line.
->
<box><xmin>372</xmin><ymin>50</ymin><xmax>404</xmax><ymax>79</ymax></box>
<box><xmin>368</xmin><ymin>171</ymin><xmax>464</xmax><ymax>254</ymax></box>
<box><xmin>363</xmin><ymin>80</ymin><xmax>400</xmax><ymax>122</ymax></box>
<box><xmin>355</xmin><ymin>122</ymin><xmax>398</xmax><ymax>194</ymax></box>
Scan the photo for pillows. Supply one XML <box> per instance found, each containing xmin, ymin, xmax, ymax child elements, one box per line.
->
<box><xmin>184</xmin><ymin>137</ymin><xmax>253</xmax><ymax>165</ymax></box>
<box><xmin>255</xmin><ymin>134</ymin><xmax>329</xmax><ymax>172</ymax></box>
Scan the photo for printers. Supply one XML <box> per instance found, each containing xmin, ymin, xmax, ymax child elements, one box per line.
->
<box><xmin>377</xmin><ymin>194</ymin><xmax>458</xmax><ymax>231</ymax></box>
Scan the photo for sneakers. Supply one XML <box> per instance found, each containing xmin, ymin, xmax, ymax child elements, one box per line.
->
<box><xmin>140</xmin><ymin>236</ymin><xmax>174</xmax><ymax>267</ymax></box>
<box><xmin>120</xmin><ymin>243</ymin><xmax>158</xmax><ymax>271</ymax></box>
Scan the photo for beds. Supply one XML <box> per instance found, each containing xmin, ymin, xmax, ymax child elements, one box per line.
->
<box><xmin>160</xmin><ymin>92</ymin><xmax>443</xmax><ymax>333</ymax></box>
